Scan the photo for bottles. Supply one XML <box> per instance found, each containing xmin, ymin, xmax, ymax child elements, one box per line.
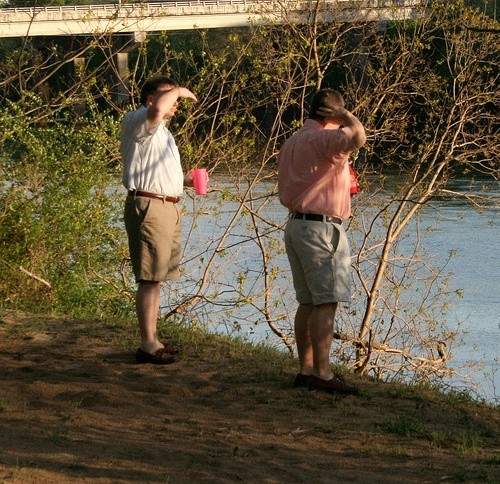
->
<box><xmin>348</xmin><ymin>158</ymin><xmax>358</xmax><ymax>194</ymax></box>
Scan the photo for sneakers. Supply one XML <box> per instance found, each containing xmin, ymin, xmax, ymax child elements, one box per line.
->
<box><xmin>307</xmin><ymin>370</ymin><xmax>359</xmax><ymax>395</ymax></box>
<box><xmin>294</xmin><ymin>372</ymin><xmax>314</xmax><ymax>388</ymax></box>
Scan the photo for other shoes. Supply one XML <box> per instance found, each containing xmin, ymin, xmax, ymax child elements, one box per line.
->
<box><xmin>135</xmin><ymin>346</ymin><xmax>176</xmax><ymax>365</ymax></box>
<box><xmin>156</xmin><ymin>342</ymin><xmax>179</xmax><ymax>354</ymax></box>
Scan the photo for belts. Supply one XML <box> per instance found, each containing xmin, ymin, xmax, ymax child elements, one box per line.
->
<box><xmin>127</xmin><ymin>189</ymin><xmax>181</xmax><ymax>204</ymax></box>
<box><xmin>291</xmin><ymin>212</ymin><xmax>342</xmax><ymax>226</ymax></box>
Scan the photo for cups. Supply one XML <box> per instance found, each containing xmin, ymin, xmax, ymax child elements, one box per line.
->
<box><xmin>192</xmin><ymin>169</ymin><xmax>207</xmax><ymax>196</ymax></box>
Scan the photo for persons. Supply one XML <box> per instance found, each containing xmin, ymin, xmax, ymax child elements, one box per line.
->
<box><xmin>278</xmin><ymin>89</ymin><xmax>366</xmax><ymax>393</ymax></box>
<box><xmin>121</xmin><ymin>77</ymin><xmax>209</xmax><ymax>364</ymax></box>
